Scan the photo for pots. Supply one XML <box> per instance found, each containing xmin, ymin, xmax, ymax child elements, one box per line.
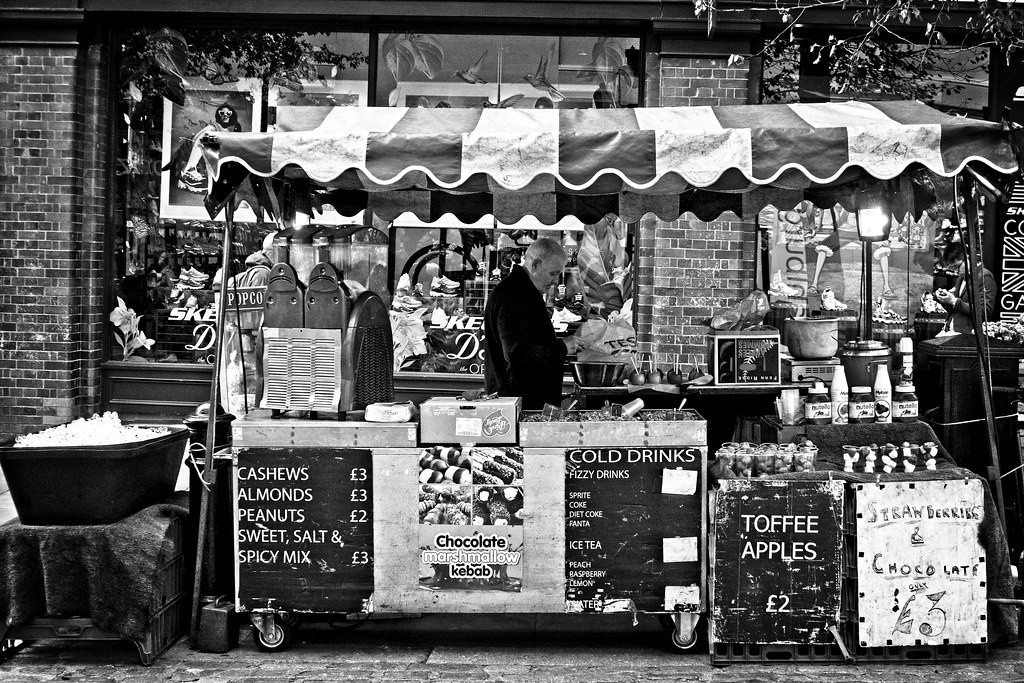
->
<box><xmin>784</xmin><ymin>316</ymin><xmax>838</xmax><ymax>359</ymax></box>
<box><xmin>842</xmin><ymin>340</ymin><xmax>893</xmax><ymax>387</ymax></box>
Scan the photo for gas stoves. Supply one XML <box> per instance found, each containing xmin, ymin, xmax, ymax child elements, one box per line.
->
<box><xmin>782</xmin><ymin>357</ymin><xmax>840</xmax><ymax>382</ymax></box>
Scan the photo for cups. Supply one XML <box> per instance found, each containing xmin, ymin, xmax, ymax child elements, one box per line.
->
<box><xmin>862</xmin><ymin>447</ymin><xmax>879</xmax><ymax>474</ymax></box>
<box><xmin>880</xmin><ymin>445</ymin><xmax>899</xmax><ymax>473</ymax></box>
<box><xmin>721</xmin><ymin>441</ymin><xmax>819</xmax><ymax>475</ymax></box>
<box><xmin>842</xmin><ymin>446</ymin><xmax>860</xmax><ymax>472</ymax></box>
<box><xmin>901</xmin><ymin>446</ymin><xmax>920</xmax><ymax>473</ymax></box>
<box><xmin>921</xmin><ymin>446</ymin><xmax>939</xmax><ymax>470</ymax></box>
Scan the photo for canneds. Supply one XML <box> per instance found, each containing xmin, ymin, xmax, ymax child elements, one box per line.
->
<box><xmin>892</xmin><ymin>386</ymin><xmax>918</xmax><ymax>422</ymax></box>
<box><xmin>805</xmin><ymin>388</ymin><xmax>832</xmax><ymax>425</ymax></box>
<box><xmin>849</xmin><ymin>386</ymin><xmax>875</xmax><ymax>423</ymax></box>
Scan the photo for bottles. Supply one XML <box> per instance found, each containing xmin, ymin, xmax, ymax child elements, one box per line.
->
<box><xmin>875</xmin><ymin>365</ymin><xmax>892</xmax><ymax>424</ymax></box>
<box><xmin>804</xmin><ymin>388</ymin><xmax>832</xmax><ymax>425</ymax></box>
<box><xmin>893</xmin><ymin>385</ymin><xmax>919</xmax><ymax>421</ymax></box>
<box><xmin>831</xmin><ymin>365</ymin><xmax>850</xmax><ymax>426</ymax></box>
<box><xmin>850</xmin><ymin>386</ymin><xmax>875</xmax><ymax>422</ymax></box>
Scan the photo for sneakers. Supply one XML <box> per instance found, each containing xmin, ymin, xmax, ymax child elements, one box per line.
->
<box><xmin>551</xmin><ymin>306</ymin><xmax>582</xmax><ymax>322</ymax></box>
<box><xmin>450</xmin><ymin>307</ymin><xmax>469</xmax><ymax>318</ymax></box>
<box><xmin>176</xmin><ymin>276</ymin><xmax>206</xmax><ymax>289</ymax></box>
<box><xmin>431</xmin><ymin>274</ymin><xmax>460</xmax><ymax>289</ymax></box>
<box><xmin>476</xmin><ymin>260</ymin><xmax>488</xmax><ymax>276</ymax></box>
<box><xmin>920</xmin><ymin>289</ymin><xmax>948</xmax><ymax>313</ymax></box>
<box><xmin>392</xmin><ymin>294</ymin><xmax>422</xmax><ymax>309</ymax></box>
<box><xmin>934</xmin><ymin>231</ymin><xmax>950</xmax><ymax>244</ymax></box>
<box><xmin>554</xmin><ymin>283</ymin><xmax>569</xmax><ymax>302</ymax></box>
<box><xmin>821</xmin><ymin>285</ymin><xmax>848</xmax><ymax>311</ymax></box>
<box><xmin>183</xmin><ymin>295</ymin><xmax>199</xmax><ymax>310</ymax></box>
<box><xmin>466</xmin><ymin>306</ymin><xmax>485</xmax><ymax>317</ymax></box>
<box><xmin>178</xmin><ymin>265</ymin><xmax>209</xmax><ymax>281</ymax></box>
<box><xmin>396</xmin><ymin>272</ymin><xmax>411</xmax><ymax>291</ymax></box>
<box><xmin>167</xmin><ymin>288</ymin><xmax>184</xmax><ymax>304</ymax></box>
<box><xmin>573</xmin><ymin>292</ymin><xmax>584</xmax><ymax>307</ymax></box>
<box><xmin>872</xmin><ymin>305</ymin><xmax>908</xmax><ymax>325</ymax></box>
<box><xmin>489</xmin><ymin>268</ymin><xmax>501</xmax><ymax>281</ymax></box>
<box><xmin>429</xmin><ymin>284</ymin><xmax>461</xmax><ymax>297</ymax></box>
<box><xmin>177</xmin><ymin>167</ymin><xmax>207</xmax><ymax>193</ymax></box>
<box><xmin>411</xmin><ymin>283</ymin><xmax>424</xmax><ymax>297</ymax></box>
<box><xmin>128</xmin><ymin>259</ymin><xmax>145</xmax><ymax>274</ymax></box>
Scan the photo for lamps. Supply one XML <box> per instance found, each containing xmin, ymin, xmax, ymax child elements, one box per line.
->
<box><xmin>853</xmin><ymin>168</ymin><xmax>893</xmax><ymax>244</ymax></box>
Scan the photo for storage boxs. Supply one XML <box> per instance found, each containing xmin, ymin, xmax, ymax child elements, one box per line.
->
<box><xmin>707</xmin><ymin>324</ymin><xmax>783</xmax><ymax>386</ymax></box>
<box><xmin>418</xmin><ymin>396</ymin><xmax>523</xmax><ymax>449</ymax></box>
<box><xmin>519</xmin><ymin>408</ymin><xmax>708</xmax><ymax>446</ymax></box>
<box><xmin>0</xmin><ymin>414</ymin><xmax>190</xmax><ymax>526</ymax></box>
<box><xmin>0</xmin><ymin>490</ymin><xmax>195</xmax><ymax>666</ymax></box>
<box><xmin>231</xmin><ymin>409</ymin><xmax>420</xmax><ymax>448</ymax></box>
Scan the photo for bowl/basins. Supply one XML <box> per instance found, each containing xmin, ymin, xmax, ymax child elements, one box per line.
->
<box><xmin>571</xmin><ymin>360</ymin><xmax>626</xmax><ymax>386</ymax></box>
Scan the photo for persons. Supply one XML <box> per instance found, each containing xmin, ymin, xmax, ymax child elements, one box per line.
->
<box><xmin>933</xmin><ymin>243</ymin><xmax>998</xmax><ymax>339</ymax></box>
<box><xmin>482</xmin><ymin>237</ymin><xmax>584</xmax><ymax>410</ymax></box>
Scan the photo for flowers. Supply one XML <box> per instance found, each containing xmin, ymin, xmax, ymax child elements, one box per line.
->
<box><xmin>109</xmin><ymin>295</ymin><xmax>155</xmax><ymax>361</ymax></box>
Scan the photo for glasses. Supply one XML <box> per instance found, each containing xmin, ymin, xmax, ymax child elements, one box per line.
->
<box><xmin>949</xmin><ymin>261</ymin><xmax>964</xmax><ymax>273</ymax></box>
<box><xmin>218</xmin><ymin>110</ymin><xmax>232</xmax><ymax>117</ymax></box>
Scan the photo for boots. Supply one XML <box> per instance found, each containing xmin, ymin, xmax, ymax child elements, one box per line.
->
<box><xmin>420</xmin><ymin>565</ymin><xmax>449</xmax><ymax>589</ymax></box>
<box><xmin>767</xmin><ymin>269</ymin><xmax>800</xmax><ymax>298</ymax></box>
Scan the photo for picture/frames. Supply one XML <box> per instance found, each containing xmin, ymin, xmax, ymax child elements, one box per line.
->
<box><xmin>160</xmin><ymin>74</ymin><xmax>265</xmax><ymax>223</ymax></box>
<box><xmin>396</xmin><ymin>80</ymin><xmax>604</xmax><ymax>112</ymax></box>
<box><xmin>268</xmin><ymin>76</ymin><xmax>369</xmax><ymax>226</ymax></box>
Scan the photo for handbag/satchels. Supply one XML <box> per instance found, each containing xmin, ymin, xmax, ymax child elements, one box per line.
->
<box><xmin>573</xmin><ymin>316</ymin><xmax>637</xmax><ymax>362</ymax></box>
<box><xmin>710</xmin><ymin>290</ymin><xmax>771</xmax><ymax>330</ymax></box>
